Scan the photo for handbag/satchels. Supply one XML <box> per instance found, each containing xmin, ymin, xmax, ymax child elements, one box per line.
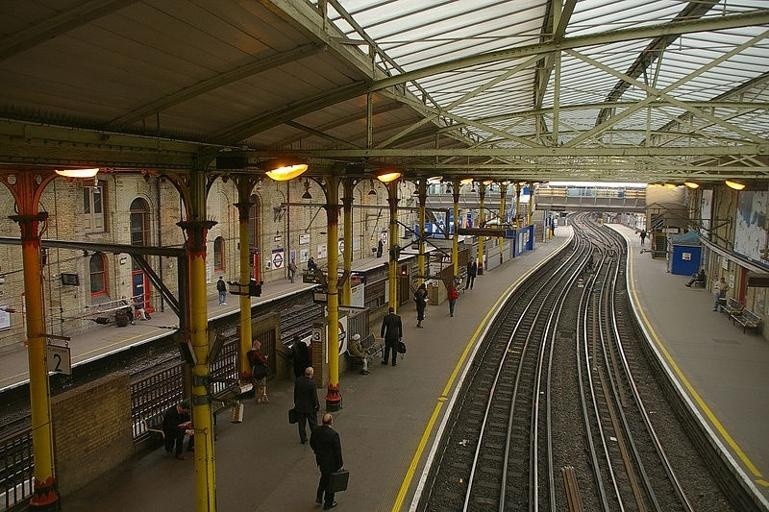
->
<box><xmin>399</xmin><ymin>342</ymin><xmax>406</xmax><ymax>353</ymax></box>
<box><xmin>254</xmin><ymin>362</ymin><xmax>270</xmax><ymax>379</ymax></box>
<box><xmin>289</xmin><ymin>408</ymin><xmax>297</xmax><ymax>424</ymax></box>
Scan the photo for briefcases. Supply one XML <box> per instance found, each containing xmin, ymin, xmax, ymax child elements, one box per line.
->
<box><xmin>331</xmin><ymin>470</ymin><xmax>350</xmax><ymax>492</ymax></box>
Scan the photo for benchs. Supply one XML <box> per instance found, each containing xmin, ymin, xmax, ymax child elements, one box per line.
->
<box><xmin>142</xmin><ymin>398</ymin><xmax>227</xmax><ymax>441</ymax></box>
<box><xmin>346</xmin><ymin>331</ymin><xmax>385</xmax><ymax>371</ymax></box>
<box><xmin>456</xmin><ymin>285</ymin><xmax>465</xmax><ymax>293</ymax></box>
<box><xmin>730</xmin><ymin>307</ymin><xmax>762</xmax><ymax>334</ymax></box>
<box><xmin>695</xmin><ymin>275</ymin><xmax>706</xmax><ymax>288</ymax></box>
<box><xmin>720</xmin><ymin>297</ymin><xmax>745</xmax><ymax>320</ymax></box>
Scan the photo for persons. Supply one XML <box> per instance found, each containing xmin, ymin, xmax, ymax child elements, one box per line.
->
<box><xmin>294</xmin><ymin>367</ymin><xmax>320</xmax><ymax>444</ymax></box>
<box><xmin>466</xmin><ymin>256</ymin><xmax>477</xmax><ymax>290</ymax></box>
<box><xmin>122</xmin><ymin>296</ymin><xmax>147</xmax><ymax>325</ymax></box>
<box><xmin>640</xmin><ymin>229</ymin><xmax>646</xmax><ymax>245</ymax></box>
<box><xmin>712</xmin><ymin>278</ymin><xmax>730</xmax><ymax>312</ymax></box>
<box><xmin>378</xmin><ymin>239</ymin><xmax>383</xmax><ymax>253</ymax></box>
<box><xmin>308</xmin><ymin>257</ymin><xmax>317</xmax><ymax>270</ymax></box>
<box><xmin>310</xmin><ymin>414</ymin><xmax>344</xmax><ymax>509</ymax></box>
<box><xmin>290</xmin><ymin>335</ymin><xmax>309</xmax><ymax>376</ymax></box>
<box><xmin>162</xmin><ymin>399</ymin><xmax>194</xmax><ymax>460</ymax></box>
<box><xmin>290</xmin><ymin>259</ymin><xmax>296</xmax><ymax>283</ymax></box>
<box><xmin>684</xmin><ymin>269</ymin><xmax>704</xmax><ymax>287</ymax></box>
<box><xmin>247</xmin><ymin>339</ymin><xmax>270</xmax><ymax>405</ymax></box>
<box><xmin>217</xmin><ymin>276</ymin><xmax>227</xmax><ymax>305</ymax></box>
<box><xmin>381</xmin><ymin>307</ymin><xmax>402</xmax><ymax>366</ymax></box>
<box><xmin>447</xmin><ymin>281</ymin><xmax>458</xmax><ymax>317</ymax></box>
<box><xmin>414</xmin><ymin>283</ymin><xmax>428</xmax><ymax>328</ymax></box>
<box><xmin>349</xmin><ymin>334</ymin><xmax>370</xmax><ymax>375</ymax></box>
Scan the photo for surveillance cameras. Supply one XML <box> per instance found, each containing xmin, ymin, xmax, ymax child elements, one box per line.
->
<box><xmin>230</xmin><ymin>403</ymin><xmax>245</xmax><ymax>424</ymax></box>
<box><xmin>232</xmin><ymin>382</ymin><xmax>254</xmax><ymax>396</ymax></box>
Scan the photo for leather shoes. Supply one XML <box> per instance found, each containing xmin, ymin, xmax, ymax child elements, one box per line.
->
<box><xmin>316</xmin><ymin>497</ymin><xmax>321</xmax><ymax>505</ymax></box>
<box><xmin>324</xmin><ymin>501</ymin><xmax>336</xmax><ymax>509</ymax></box>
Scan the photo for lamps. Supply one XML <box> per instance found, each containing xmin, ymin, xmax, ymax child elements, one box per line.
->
<box><xmin>55</xmin><ymin>168</ymin><xmax>99</xmax><ymax>178</ymax></box>
<box><xmin>725</xmin><ymin>179</ymin><xmax>747</xmax><ymax>190</ymax></box>
<box><xmin>376</xmin><ymin>168</ymin><xmax>402</xmax><ymax>182</ymax></box>
<box><xmin>302</xmin><ymin>179</ymin><xmax>312</xmax><ymax>198</ymax></box>
<box><xmin>368</xmin><ymin>179</ymin><xmax>377</xmax><ymax>194</ymax></box>
<box><xmin>648</xmin><ymin>180</ymin><xmax>700</xmax><ymax>189</ymax></box>
<box><xmin>427</xmin><ymin>176</ymin><xmax>540</xmax><ymax>193</ymax></box>
<box><xmin>263</xmin><ymin>157</ymin><xmax>309</xmax><ymax>181</ymax></box>
